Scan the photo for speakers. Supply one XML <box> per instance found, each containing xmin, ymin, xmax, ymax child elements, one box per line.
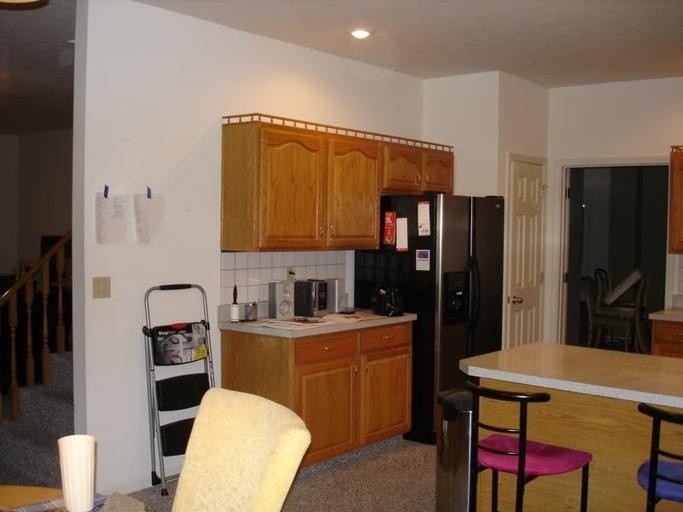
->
<box><xmin>269</xmin><ymin>279</ymin><xmax>294</xmax><ymax>320</ymax></box>
<box><xmin>325</xmin><ymin>278</ymin><xmax>345</xmax><ymax>313</ymax></box>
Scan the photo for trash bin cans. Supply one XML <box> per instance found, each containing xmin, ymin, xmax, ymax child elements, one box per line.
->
<box><xmin>435</xmin><ymin>388</ymin><xmax>476</xmax><ymax>512</ymax></box>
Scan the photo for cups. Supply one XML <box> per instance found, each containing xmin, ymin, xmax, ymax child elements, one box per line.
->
<box><xmin>56</xmin><ymin>432</ymin><xmax>96</xmax><ymax>512</ymax></box>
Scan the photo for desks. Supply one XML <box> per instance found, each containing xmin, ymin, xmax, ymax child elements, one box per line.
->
<box><xmin>0</xmin><ymin>483</ymin><xmax>63</xmax><ymax>510</ymax></box>
<box><xmin>457</xmin><ymin>342</ymin><xmax>683</xmax><ymax>413</ymax></box>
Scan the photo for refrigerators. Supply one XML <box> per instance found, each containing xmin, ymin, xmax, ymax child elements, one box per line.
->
<box><xmin>350</xmin><ymin>188</ymin><xmax>504</xmax><ymax>446</ymax></box>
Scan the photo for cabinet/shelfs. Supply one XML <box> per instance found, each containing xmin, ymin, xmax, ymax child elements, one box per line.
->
<box><xmin>220</xmin><ymin>116</ymin><xmax>383</xmax><ymax>252</ymax></box>
<box><xmin>382</xmin><ymin>132</ymin><xmax>455</xmax><ymax>190</ymax></box>
<box><xmin>650</xmin><ymin>315</ymin><xmax>683</xmax><ymax>358</ymax></box>
<box><xmin>667</xmin><ymin>144</ymin><xmax>682</xmax><ymax>253</ymax></box>
<box><xmin>220</xmin><ymin>310</ymin><xmax>413</xmax><ymax>469</ymax></box>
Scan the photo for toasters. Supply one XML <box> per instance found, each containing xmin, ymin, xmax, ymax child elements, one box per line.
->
<box><xmin>296</xmin><ymin>279</ymin><xmax>328</xmax><ymax>318</ymax></box>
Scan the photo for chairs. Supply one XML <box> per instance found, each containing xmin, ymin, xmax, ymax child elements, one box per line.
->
<box><xmin>453</xmin><ymin>371</ymin><xmax>591</xmax><ymax>510</ymax></box>
<box><xmin>579</xmin><ymin>267</ymin><xmax>648</xmax><ymax>354</ymax></box>
<box><xmin>166</xmin><ymin>387</ymin><xmax>313</xmax><ymax>511</ymax></box>
<box><xmin>637</xmin><ymin>402</ymin><xmax>681</xmax><ymax>511</ymax></box>
